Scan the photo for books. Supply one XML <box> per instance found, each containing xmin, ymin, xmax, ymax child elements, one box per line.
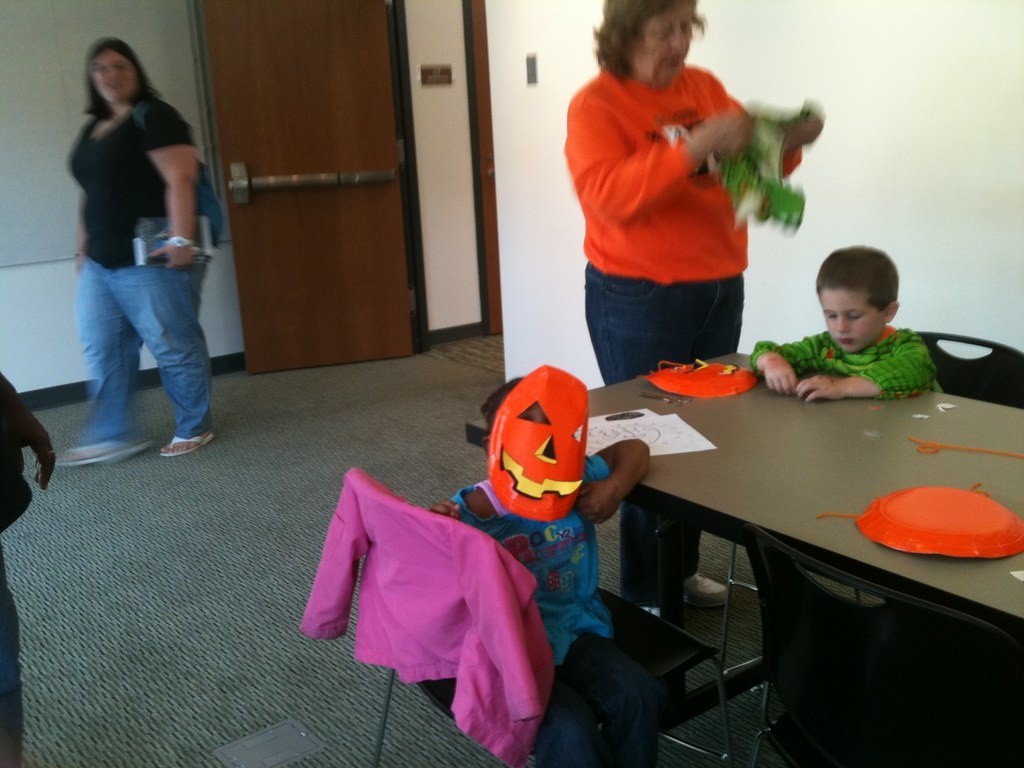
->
<box><xmin>133</xmin><ymin>215</ymin><xmax>213</xmax><ymax>268</ymax></box>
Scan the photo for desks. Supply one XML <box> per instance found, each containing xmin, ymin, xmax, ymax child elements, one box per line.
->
<box><xmin>464</xmin><ymin>352</ymin><xmax>1024</xmax><ymax>767</ymax></box>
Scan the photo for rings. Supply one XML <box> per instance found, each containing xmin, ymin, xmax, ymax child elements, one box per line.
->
<box><xmin>49</xmin><ymin>450</ymin><xmax>55</xmax><ymax>453</ymax></box>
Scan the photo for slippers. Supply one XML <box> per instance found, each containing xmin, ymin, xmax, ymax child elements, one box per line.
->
<box><xmin>57</xmin><ymin>439</ymin><xmax>152</xmax><ymax>466</ymax></box>
<box><xmin>160</xmin><ymin>433</ymin><xmax>214</xmax><ymax>457</ymax></box>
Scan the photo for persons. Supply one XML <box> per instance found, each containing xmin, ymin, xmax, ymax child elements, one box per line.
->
<box><xmin>422</xmin><ymin>376</ymin><xmax>659</xmax><ymax>767</ymax></box>
<box><xmin>56</xmin><ymin>36</ymin><xmax>215</xmax><ymax>469</ymax></box>
<box><xmin>752</xmin><ymin>246</ymin><xmax>942</xmax><ymax>402</ymax></box>
<box><xmin>0</xmin><ymin>374</ymin><xmax>60</xmax><ymax>767</ymax></box>
<box><xmin>563</xmin><ymin>0</ymin><xmax>823</xmax><ymax>632</ymax></box>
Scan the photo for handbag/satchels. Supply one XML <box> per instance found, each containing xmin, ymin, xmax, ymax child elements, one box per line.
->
<box><xmin>133</xmin><ymin>96</ymin><xmax>223</xmax><ymax>248</ymax></box>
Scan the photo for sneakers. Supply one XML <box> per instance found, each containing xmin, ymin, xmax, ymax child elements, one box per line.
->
<box><xmin>680</xmin><ymin>572</ymin><xmax>729</xmax><ymax>608</ymax></box>
<box><xmin>640</xmin><ymin>607</ymin><xmax>659</xmax><ymax>618</ymax></box>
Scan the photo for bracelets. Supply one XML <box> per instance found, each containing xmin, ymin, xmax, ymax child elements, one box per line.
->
<box><xmin>166</xmin><ymin>237</ymin><xmax>194</xmax><ymax>246</ymax></box>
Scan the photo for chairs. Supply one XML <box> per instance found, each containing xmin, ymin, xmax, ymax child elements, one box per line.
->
<box><xmin>738</xmin><ymin>519</ymin><xmax>1024</xmax><ymax>767</ymax></box>
<box><xmin>343</xmin><ymin>467</ymin><xmax>737</xmax><ymax>767</ymax></box>
<box><xmin>913</xmin><ymin>332</ymin><xmax>1024</xmax><ymax>408</ymax></box>
